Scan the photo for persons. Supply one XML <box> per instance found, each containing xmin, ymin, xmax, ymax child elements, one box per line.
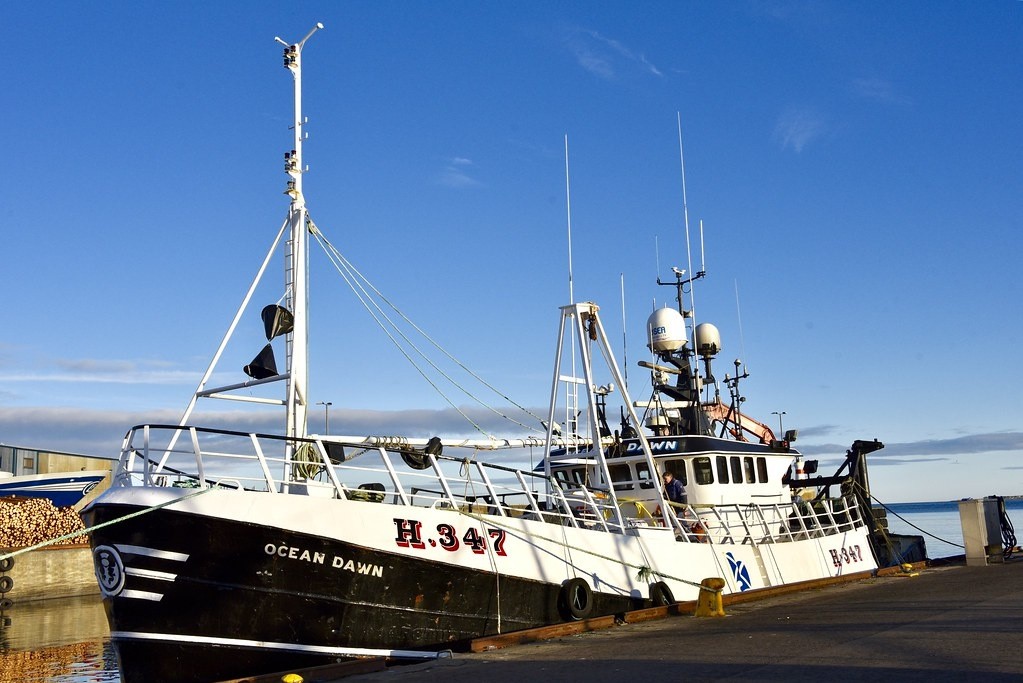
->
<box><xmin>662</xmin><ymin>472</ymin><xmax>695</xmax><ymax>541</ymax></box>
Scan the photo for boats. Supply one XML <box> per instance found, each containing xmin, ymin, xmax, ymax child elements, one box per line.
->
<box><xmin>75</xmin><ymin>18</ymin><xmax>928</xmax><ymax>677</ymax></box>
<box><xmin>525</xmin><ymin>107</ymin><xmax>806</xmax><ymax>542</ymax></box>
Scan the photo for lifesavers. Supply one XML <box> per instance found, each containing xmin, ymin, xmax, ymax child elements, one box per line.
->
<box><xmin>652</xmin><ymin>581</ymin><xmax>675</xmax><ymax>606</ymax></box>
<box><xmin>0</xmin><ymin>598</ymin><xmax>12</xmax><ymax>607</ymax></box>
<box><xmin>0</xmin><ymin>576</ymin><xmax>13</xmax><ymax>593</ymax></box>
<box><xmin>576</xmin><ymin>506</ymin><xmax>592</xmax><ymax>519</ymax></box>
<box><xmin>563</xmin><ymin>578</ymin><xmax>593</xmax><ymax>619</ymax></box>
<box><xmin>0</xmin><ymin>556</ymin><xmax>14</xmax><ymax>572</ymax></box>
